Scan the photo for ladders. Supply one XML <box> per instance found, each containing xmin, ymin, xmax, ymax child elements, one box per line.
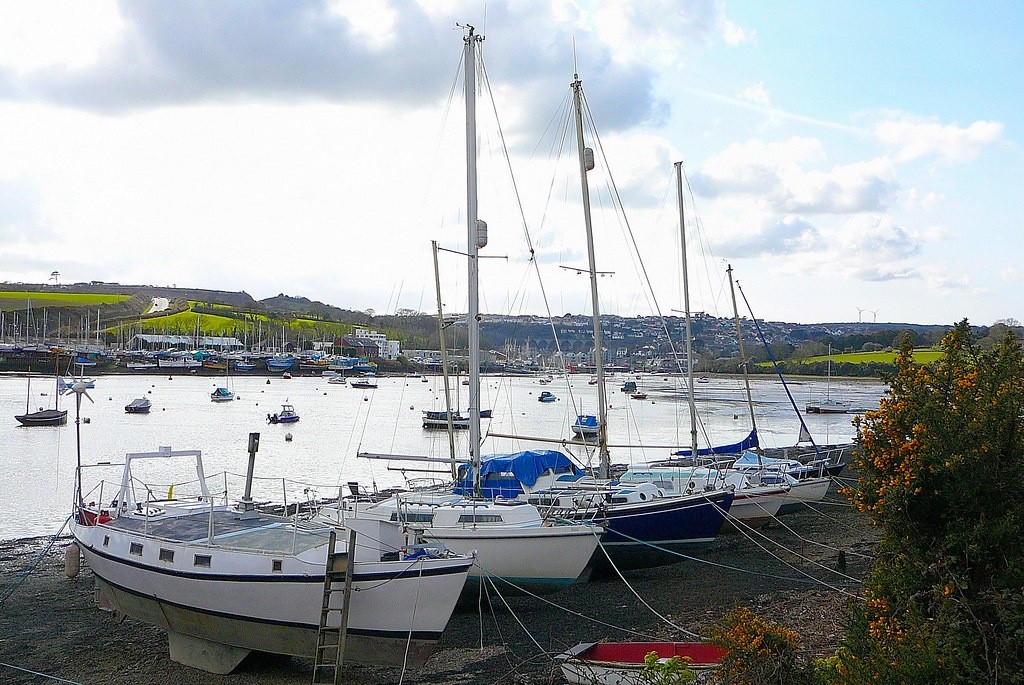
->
<box><xmin>312</xmin><ymin>529</ymin><xmax>356</xmax><ymax>685</ymax></box>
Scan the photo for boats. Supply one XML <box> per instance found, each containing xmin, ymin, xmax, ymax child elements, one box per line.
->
<box><xmin>125</xmin><ymin>399</ymin><xmax>152</xmax><ymax>414</ymax></box>
<box><xmin>408</xmin><ymin>372</ymin><xmax>421</xmax><ymax>377</ymax></box>
<box><xmin>539</xmin><ymin>391</ymin><xmax>556</xmax><ymax>403</ymax></box>
<box><xmin>267</xmin><ymin>404</ymin><xmax>301</xmax><ymax>424</ymax></box>
<box><xmin>632</xmin><ymin>391</ymin><xmax>648</xmax><ymax>400</ymax></box>
<box><xmin>554</xmin><ymin>640</ymin><xmax>731</xmax><ymax>684</ymax></box>
<box><xmin>350</xmin><ymin>378</ymin><xmax>377</xmax><ymax>389</ymax></box>
<box><xmin>478</xmin><ymin>409</ymin><xmax>491</xmax><ymax>418</ymax></box>
<box><xmin>68</xmin><ymin>443</ymin><xmax>476</xmax><ymax>675</ymax></box>
<box><xmin>620</xmin><ymin>382</ymin><xmax>636</xmax><ymax>392</ymax></box>
<box><xmin>696</xmin><ymin>378</ymin><xmax>708</xmax><ymax>383</ymax></box>
<box><xmin>570</xmin><ymin>412</ymin><xmax>600</xmax><ymax>436</ymax></box>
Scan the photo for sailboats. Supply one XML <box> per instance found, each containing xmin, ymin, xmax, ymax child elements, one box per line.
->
<box><xmin>311</xmin><ymin>22</ymin><xmax>612</xmax><ymax>590</ymax></box>
<box><xmin>420</xmin><ymin>319</ymin><xmax>470</xmax><ymax>428</ymax></box>
<box><xmin>450</xmin><ymin>76</ymin><xmax>848</xmax><ymax>564</ymax></box>
<box><xmin>807</xmin><ymin>342</ymin><xmax>851</xmax><ymax>414</ymax></box>
<box><xmin>0</xmin><ymin>295</ymin><xmax>378</xmax><ymax>384</ymax></box>
<box><xmin>14</xmin><ymin>365</ymin><xmax>67</xmax><ymax>425</ymax></box>
<box><xmin>211</xmin><ymin>353</ymin><xmax>236</xmax><ymax>402</ymax></box>
